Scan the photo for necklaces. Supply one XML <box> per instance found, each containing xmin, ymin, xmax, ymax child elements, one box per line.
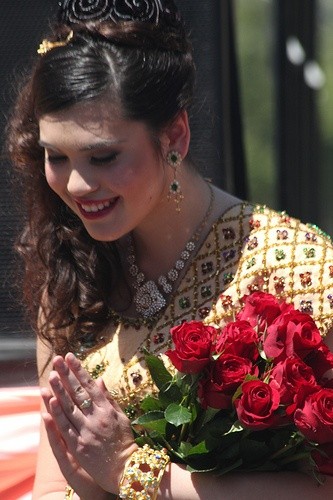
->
<box><xmin>126</xmin><ymin>176</ymin><xmax>215</xmax><ymax>319</ymax></box>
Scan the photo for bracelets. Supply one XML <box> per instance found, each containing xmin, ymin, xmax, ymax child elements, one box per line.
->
<box><xmin>65</xmin><ymin>485</ymin><xmax>74</xmax><ymax>500</ymax></box>
<box><xmin>119</xmin><ymin>443</ymin><xmax>170</xmax><ymax>500</ymax></box>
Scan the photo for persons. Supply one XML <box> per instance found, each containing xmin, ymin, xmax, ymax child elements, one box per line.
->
<box><xmin>6</xmin><ymin>0</ymin><xmax>333</xmax><ymax>500</ymax></box>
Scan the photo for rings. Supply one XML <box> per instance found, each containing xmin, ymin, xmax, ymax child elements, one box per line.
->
<box><xmin>79</xmin><ymin>398</ymin><xmax>92</xmax><ymax>410</ymax></box>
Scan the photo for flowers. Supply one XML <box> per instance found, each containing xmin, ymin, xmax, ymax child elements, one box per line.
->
<box><xmin>124</xmin><ymin>290</ymin><xmax>333</xmax><ymax>487</ymax></box>
